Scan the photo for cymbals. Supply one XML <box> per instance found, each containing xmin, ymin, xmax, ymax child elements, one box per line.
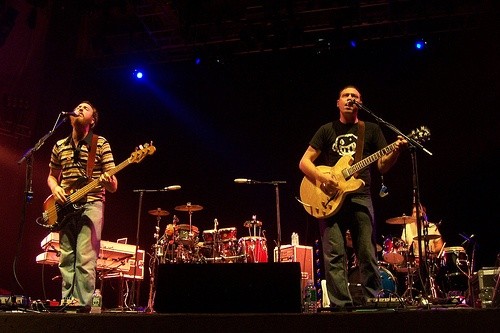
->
<box><xmin>386</xmin><ymin>216</ymin><xmax>416</xmax><ymax>224</ymax></box>
<box><xmin>148</xmin><ymin>210</ymin><xmax>170</xmax><ymax>216</ymax></box>
<box><xmin>174</xmin><ymin>205</ymin><xmax>203</xmax><ymax>211</ymax></box>
<box><xmin>413</xmin><ymin>234</ymin><xmax>441</xmax><ymax>240</ymax></box>
<box><xmin>345</xmin><ymin>232</ymin><xmax>382</xmax><ymax>253</ymax></box>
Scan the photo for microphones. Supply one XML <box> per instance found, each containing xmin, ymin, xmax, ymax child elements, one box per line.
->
<box><xmin>234</xmin><ymin>178</ymin><xmax>252</xmax><ymax>183</ymax></box>
<box><xmin>61</xmin><ymin>110</ymin><xmax>79</xmax><ymax>117</ymax></box>
<box><xmin>347</xmin><ymin>99</ymin><xmax>353</xmax><ymax>107</ymax></box>
<box><xmin>164</xmin><ymin>185</ymin><xmax>181</xmax><ymax>191</ymax></box>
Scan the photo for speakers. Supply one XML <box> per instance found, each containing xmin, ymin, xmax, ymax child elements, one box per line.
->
<box><xmin>155</xmin><ymin>262</ymin><xmax>303</xmax><ymax>313</ymax></box>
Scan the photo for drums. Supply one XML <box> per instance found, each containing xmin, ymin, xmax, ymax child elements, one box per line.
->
<box><xmin>392</xmin><ymin>254</ymin><xmax>416</xmax><ymax>272</ymax></box>
<box><xmin>237</xmin><ymin>236</ymin><xmax>268</xmax><ymax>262</ymax></box>
<box><xmin>431</xmin><ymin>247</ymin><xmax>470</xmax><ymax>292</ymax></box>
<box><xmin>383</xmin><ymin>237</ymin><xmax>407</xmax><ymax>264</ymax></box>
<box><xmin>174</xmin><ymin>225</ymin><xmax>198</xmax><ymax>243</ymax></box>
<box><xmin>203</xmin><ymin>230</ymin><xmax>217</xmax><ymax>243</ymax></box>
<box><xmin>348</xmin><ymin>262</ymin><xmax>397</xmax><ymax>306</ymax></box>
<box><xmin>218</xmin><ymin>228</ymin><xmax>237</xmax><ymax>245</ymax></box>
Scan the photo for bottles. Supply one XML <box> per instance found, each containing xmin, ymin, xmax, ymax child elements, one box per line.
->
<box><xmin>91</xmin><ymin>289</ymin><xmax>102</xmax><ymax>314</ymax></box>
<box><xmin>305</xmin><ymin>280</ymin><xmax>317</xmax><ymax>313</ymax></box>
<box><xmin>292</xmin><ymin>232</ymin><xmax>299</xmax><ymax>246</ymax></box>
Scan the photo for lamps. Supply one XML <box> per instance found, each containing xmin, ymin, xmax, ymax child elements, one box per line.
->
<box><xmin>416</xmin><ymin>38</ymin><xmax>432</xmax><ymax>51</ymax></box>
<box><xmin>132</xmin><ymin>68</ymin><xmax>144</xmax><ymax>79</ymax></box>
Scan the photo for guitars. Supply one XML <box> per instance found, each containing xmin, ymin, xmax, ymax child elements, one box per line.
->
<box><xmin>40</xmin><ymin>143</ymin><xmax>156</xmax><ymax>231</ymax></box>
<box><xmin>300</xmin><ymin>126</ymin><xmax>431</xmax><ymax>219</ymax></box>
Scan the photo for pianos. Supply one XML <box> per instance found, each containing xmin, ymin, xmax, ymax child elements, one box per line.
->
<box><xmin>40</xmin><ymin>232</ymin><xmax>137</xmax><ymax>261</ymax></box>
<box><xmin>36</xmin><ymin>252</ymin><xmax>130</xmax><ymax>310</ymax></box>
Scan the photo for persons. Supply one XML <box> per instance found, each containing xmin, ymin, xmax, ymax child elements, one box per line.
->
<box><xmin>300</xmin><ymin>86</ymin><xmax>408</xmax><ymax>310</ymax></box>
<box><xmin>47</xmin><ymin>101</ymin><xmax>118</xmax><ymax>306</ymax></box>
<box><xmin>402</xmin><ymin>203</ymin><xmax>445</xmax><ymax>262</ymax></box>
